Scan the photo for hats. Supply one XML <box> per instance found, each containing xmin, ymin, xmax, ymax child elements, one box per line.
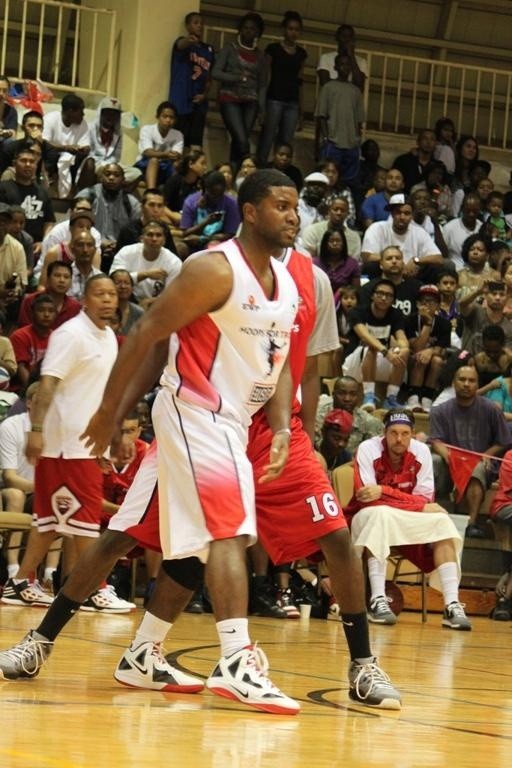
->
<box><xmin>304</xmin><ymin>172</ymin><xmax>330</xmax><ymax>186</ymax></box>
<box><xmin>100</xmin><ymin>98</ymin><xmax>124</xmax><ymax>114</ymax></box>
<box><xmin>324</xmin><ymin>408</ymin><xmax>353</xmax><ymax>433</ymax></box>
<box><xmin>70</xmin><ymin>211</ymin><xmax>97</xmax><ymax>227</ymax></box>
<box><xmin>0</xmin><ymin>203</ymin><xmax>15</xmax><ymax>217</ymax></box>
<box><xmin>384</xmin><ymin>194</ymin><xmax>414</xmax><ymax>211</ymax></box>
<box><xmin>420</xmin><ymin>285</ymin><xmax>440</xmax><ymax>296</ymax></box>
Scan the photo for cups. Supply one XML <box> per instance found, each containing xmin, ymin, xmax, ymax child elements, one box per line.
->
<box><xmin>299</xmin><ymin>604</ymin><xmax>312</xmax><ymax>620</ymax></box>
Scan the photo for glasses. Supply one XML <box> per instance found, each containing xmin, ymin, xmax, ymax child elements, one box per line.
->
<box><xmin>373</xmin><ymin>291</ymin><xmax>394</xmax><ymax>301</ymax></box>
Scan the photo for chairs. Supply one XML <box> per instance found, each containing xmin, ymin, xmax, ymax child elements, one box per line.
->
<box><xmin>332</xmin><ymin>460</ymin><xmax>429</xmax><ymax>624</ymax></box>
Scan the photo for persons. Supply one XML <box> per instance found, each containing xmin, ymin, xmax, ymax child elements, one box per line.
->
<box><xmin>2</xmin><ymin>75</ymin><xmax>512</xmax><ymax>638</ymax></box>
<box><xmin>254</xmin><ymin>10</ymin><xmax>309</xmax><ymax>168</ymax></box>
<box><xmin>0</xmin><ymin>242</ymin><xmax>411</xmax><ymax>711</ymax></box>
<box><xmin>167</xmin><ymin>12</ymin><xmax>215</xmax><ymax>152</ymax></box>
<box><xmin>312</xmin><ymin>52</ymin><xmax>367</xmax><ymax>185</ymax></box>
<box><xmin>76</xmin><ymin>163</ymin><xmax>318</xmax><ymax>717</ymax></box>
<box><xmin>209</xmin><ymin>12</ymin><xmax>269</xmax><ymax>176</ymax></box>
<box><xmin>312</xmin><ymin>22</ymin><xmax>372</xmax><ymax>162</ymax></box>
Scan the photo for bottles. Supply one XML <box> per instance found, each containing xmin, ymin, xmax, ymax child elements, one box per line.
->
<box><xmin>148</xmin><ymin>273</ymin><xmax>166</xmax><ymax>308</ymax></box>
<box><xmin>4</xmin><ymin>270</ymin><xmax>18</xmax><ymax>291</ymax></box>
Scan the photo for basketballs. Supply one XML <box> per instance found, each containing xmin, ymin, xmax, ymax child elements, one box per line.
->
<box><xmin>366</xmin><ymin>580</ymin><xmax>403</xmax><ymax>616</ymax></box>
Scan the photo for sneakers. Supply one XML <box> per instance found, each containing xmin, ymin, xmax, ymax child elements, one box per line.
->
<box><xmin>367</xmin><ymin>595</ymin><xmax>397</xmax><ymax>624</ymax></box>
<box><xmin>1</xmin><ymin>565</ymin><xmax>136</xmax><ymax>613</ymax></box>
<box><xmin>348</xmin><ymin>657</ymin><xmax>401</xmax><ymax>710</ymax></box>
<box><xmin>144</xmin><ymin>579</ymin><xmax>341</xmax><ymax>621</ymax></box>
<box><xmin>205</xmin><ymin>646</ymin><xmax>300</xmax><ymax>715</ymax></box>
<box><xmin>493</xmin><ymin>598</ymin><xmax>510</xmax><ymax>621</ymax></box>
<box><xmin>361</xmin><ymin>394</ymin><xmax>432</xmax><ymax>414</ymax></box>
<box><xmin>442</xmin><ymin>601</ymin><xmax>471</xmax><ymax>629</ymax></box>
<box><xmin>0</xmin><ymin>630</ymin><xmax>52</xmax><ymax>677</ymax></box>
<box><xmin>467</xmin><ymin>524</ymin><xmax>486</xmax><ymax>537</ymax></box>
<box><xmin>115</xmin><ymin>642</ymin><xmax>205</xmax><ymax>694</ymax></box>
